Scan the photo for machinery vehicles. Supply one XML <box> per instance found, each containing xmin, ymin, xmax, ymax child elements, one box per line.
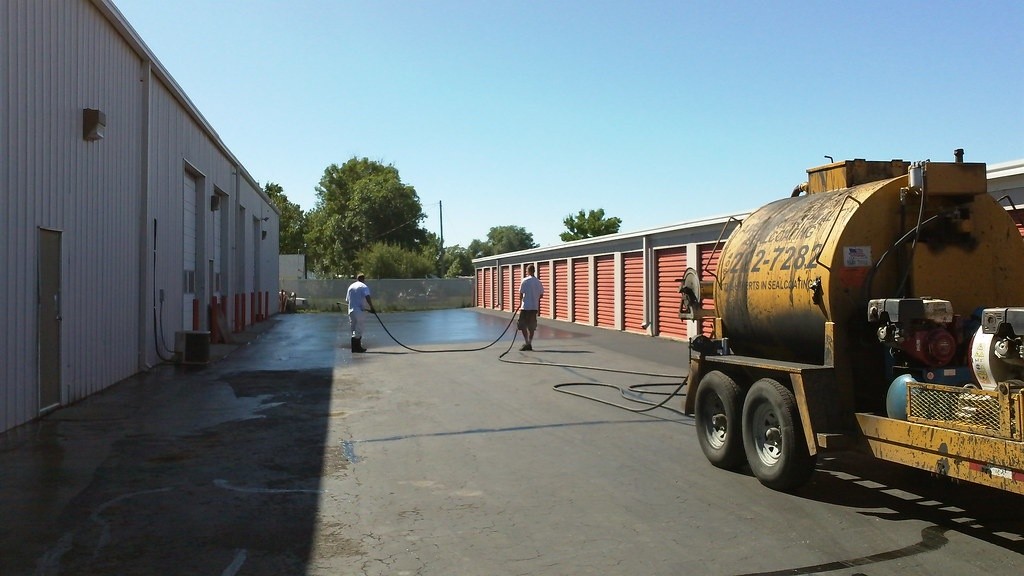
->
<box><xmin>673</xmin><ymin>149</ymin><xmax>1024</xmax><ymax>496</ymax></box>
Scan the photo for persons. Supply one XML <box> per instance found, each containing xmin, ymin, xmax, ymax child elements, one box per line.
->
<box><xmin>517</xmin><ymin>264</ymin><xmax>544</xmax><ymax>352</ymax></box>
<box><xmin>346</xmin><ymin>274</ymin><xmax>374</xmax><ymax>353</ymax></box>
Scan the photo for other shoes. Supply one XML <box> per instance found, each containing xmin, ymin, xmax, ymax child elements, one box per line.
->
<box><xmin>519</xmin><ymin>345</ymin><xmax>533</xmax><ymax>351</ymax></box>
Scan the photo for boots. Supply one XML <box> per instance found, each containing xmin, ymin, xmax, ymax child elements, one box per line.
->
<box><xmin>351</xmin><ymin>337</ymin><xmax>368</xmax><ymax>353</ymax></box>
